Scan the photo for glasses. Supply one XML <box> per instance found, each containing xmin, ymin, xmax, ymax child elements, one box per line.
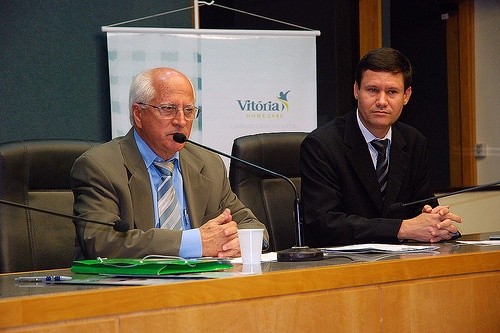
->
<box><xmin>138</xmin><ymin>102</ymin><xmax>201</xmax><ymax>121</ymax></box>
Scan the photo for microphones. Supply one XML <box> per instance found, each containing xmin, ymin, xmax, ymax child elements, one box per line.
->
<box><xmin>390</xmin><ymin>180</ymin><xmax>500</xmax><ymax>214</ymax></box>
<box><xmin>0</xmin><ymin>199</ymin><xmax>130</xmax><ymax>232</ymax></box>
<box><xmin>172</xmin><ymin>132</ymin><xmax>323</xmax><ymax>262</ymax></box>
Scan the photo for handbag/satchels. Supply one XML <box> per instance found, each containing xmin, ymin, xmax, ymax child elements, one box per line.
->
<box><xmin>70</xmin><ymin>254</ymin><xmax>234</xmax><ymax>276</ymax></box>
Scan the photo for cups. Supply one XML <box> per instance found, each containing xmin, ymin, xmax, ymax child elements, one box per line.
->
<box><xmin>237</xmin><ymin>229</ymin><xmax>265</xmax><ymax>264</ymax></box>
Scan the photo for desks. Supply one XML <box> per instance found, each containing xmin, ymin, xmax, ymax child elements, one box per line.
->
<box><xmin>0</xmin><ymin>232</ymin><xmax>500</xmax><ymax>333</ymax></box>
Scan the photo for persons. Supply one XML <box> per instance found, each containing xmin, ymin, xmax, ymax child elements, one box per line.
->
<box><xmin>69</xmin><ymin>67</ymin><xmax>269</xmax><ymax>258</ymax></box>
<box><xmin>301</xmin><ymin>47</ymin><xmax>463</xmax><ymax>248</ymax></box>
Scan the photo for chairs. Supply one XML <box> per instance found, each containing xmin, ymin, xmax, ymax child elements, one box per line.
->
<box><xmin>0</xmin><ymin>137</ymin><xmax>111</xmax><ymax>276</ymax></box>
<box><xmin>229</xmin><ymin>132</ymin><xmax>314</xmax><ymax>253</ymax></box>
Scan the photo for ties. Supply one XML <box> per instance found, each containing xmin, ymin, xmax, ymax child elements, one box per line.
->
<box><xmin>153</xmin><ymin>158</ymin><xmax>184</xmax><ymax>232</ymax></box>
<box><xmin>369</xmin><ymin>138</ymin><xmax>391</xmax><ymax>200</ymax></box>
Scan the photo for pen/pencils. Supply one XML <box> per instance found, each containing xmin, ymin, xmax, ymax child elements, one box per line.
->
<box><xmin>12</xmin><ymin>277</ymin><xmax>73</xmax><ymax>280</ymax></box>
